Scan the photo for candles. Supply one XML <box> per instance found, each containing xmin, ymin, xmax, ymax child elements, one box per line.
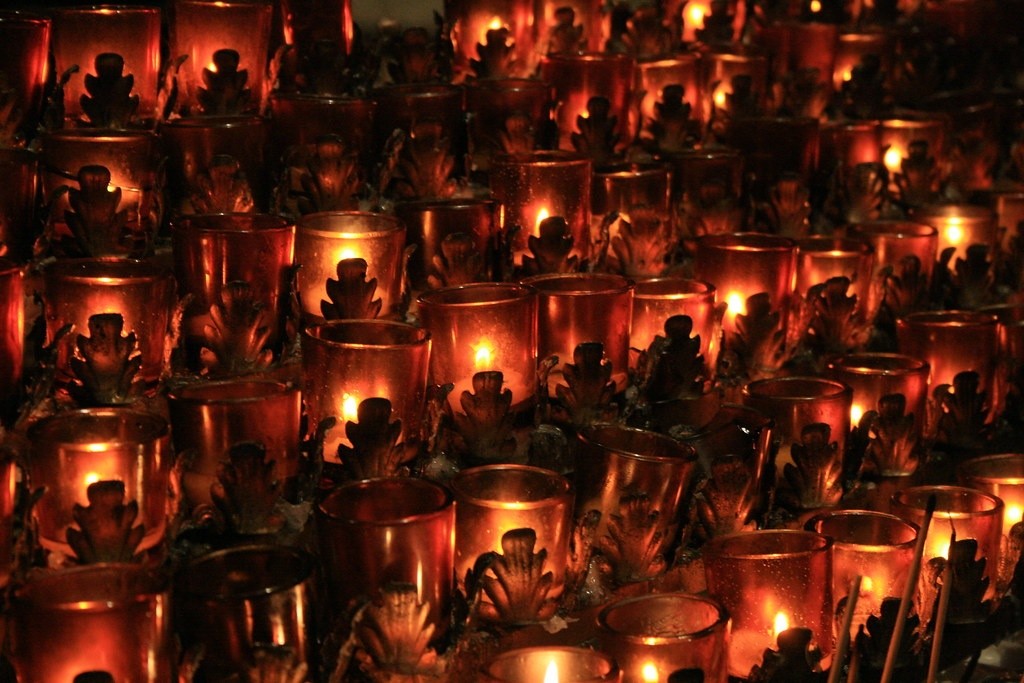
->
<box><xmin>0</xmin><ymin>0</ymin><xmax>1024</xmax><ymax>683</ymax></box>
